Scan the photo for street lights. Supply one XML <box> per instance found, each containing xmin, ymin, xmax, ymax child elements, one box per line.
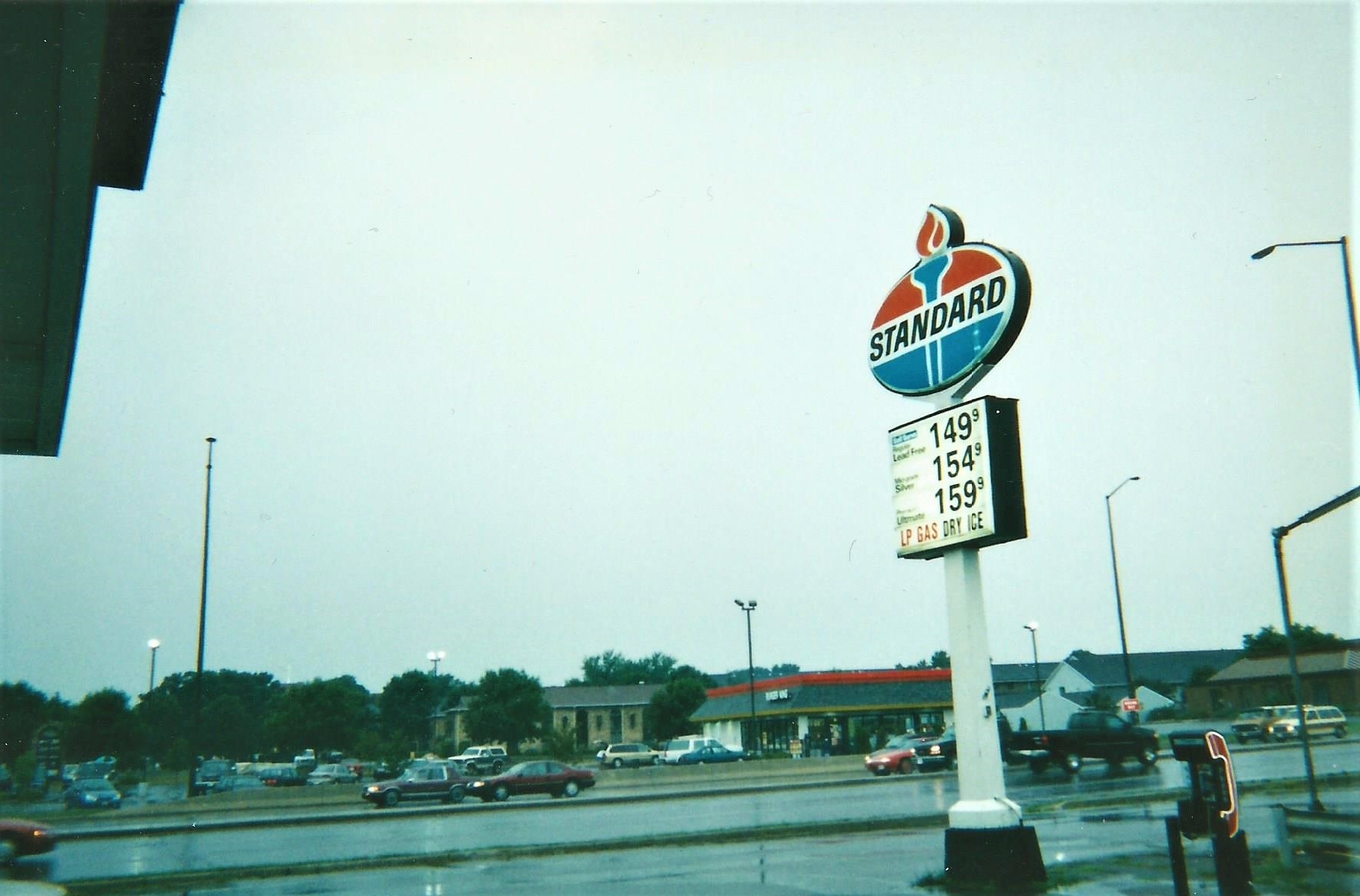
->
<box><xmin>1106</xmin><ymin>476</ymin><xmax>1142</xmax><ymax>726</ymax></box>
<box><xmin>1024</xmin><ymin>623</ymin><xmax>1046</xmax><ymax>729</ymax></box>
<box><xmin>189</xmin><ymin>438</ymin><xmax>218</xmax><ymax>798</ymax></box>
<box><xmin>427</xmin><ymin>651</ymin><xmax>446</xmax><ymax>748</ymax></box>
<box><xmin>734</xmin><ymin>599</ymin><xmax>758</xmax><ymax>750</ymax></box>
<box><xmin>146</xmin><ymin>639</ymin><xmax>159</xmax><ymax>692</ymax></box>
<box><xmin>1250</xmin><ymin>235</ymin><xmax>1360</xmax><ymax>389</ymax></box>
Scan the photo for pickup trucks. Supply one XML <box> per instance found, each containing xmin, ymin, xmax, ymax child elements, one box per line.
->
<box><xmin>1013</xmin><ymin>708</ymin><xmax>1160</xmax><ymax>775</ymax></box>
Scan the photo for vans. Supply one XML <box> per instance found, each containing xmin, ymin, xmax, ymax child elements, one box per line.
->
<box><xmin>1230</xmin><ymin>704</ymin><xmax>1350</xmax><ymax>744</ymax></box>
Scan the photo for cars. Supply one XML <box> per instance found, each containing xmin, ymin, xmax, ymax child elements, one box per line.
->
<box><xmin>659</xmin><ymin>735</ymin><xmax>751</xmax><ymax>765</ymax></box>
<box><xmin>863</xmin><ymin>708</ymin><xmax>1016</xmax><ymax>778</ymax></box>
<box><xmin>63</xmin><ymin>761</ymin><xmax>123</xmax><ymax>810</ymax></box>
<box><xmin>592</xmin><ymin>740</ymin><xmax>660</xmax><ymax>769</ymax></box>
<box><xmin>466</xmin><ymin>760</ymin><xmax>597</xmax><ymax>802</ymax></box>
<box><xmin>195</xmin><ymin>748</ymin><xmax>362</xmax><ymax>795</ymax></box>
<box><xmin>0</xmin><ymin>819</ymin><xmax>56</xmax><ymax>867</ymax></box>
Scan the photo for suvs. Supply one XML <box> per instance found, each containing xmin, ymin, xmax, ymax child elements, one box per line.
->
<box><xmin>446</xmin><ymin>746</ymin><xmax>510</xmax><ymax>777</ymax></box>
<box><xmin>362</xmin><ymin>755</ymin><xmax>471</xmax><ymax>808</ymax></box>
